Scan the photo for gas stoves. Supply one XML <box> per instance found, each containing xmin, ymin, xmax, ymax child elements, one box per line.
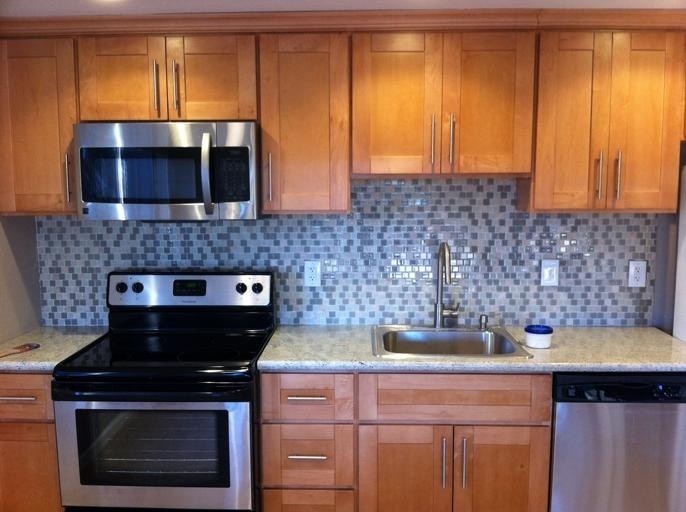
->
<box><xmin>52</xmin><ymin>268</ymin><xmax>276</xmax><ymax>374</ymax></box>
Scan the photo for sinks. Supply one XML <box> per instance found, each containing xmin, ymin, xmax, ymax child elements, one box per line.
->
<box><xmin>372</xmin><ymin>323</ymin><xmax>534</xmax><ymax>360</ymax></box>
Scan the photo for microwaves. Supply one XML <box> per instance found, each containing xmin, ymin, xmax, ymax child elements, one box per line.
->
<box><xmin>71</xmin><ymin>121</ymin><xmax>261</xmax><ymax>221</ymax></box>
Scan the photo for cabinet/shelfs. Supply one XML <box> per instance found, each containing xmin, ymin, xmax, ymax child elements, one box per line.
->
<box><xmin>262</xmin><ymin>422</ymin><xmax>355</xmax><ymax>488</ymax></box>
<box><xmin>257</xmin><ymin>33</ymin><xmax>350</xmax><ymax>216</ymax></box>
<box><xmin>535</xmin><ymin>26</ymin><xmax>686</xmax><ymax>219</ymax></box>
<box><xmin>264</xmin><ymin>488</ymin><xmax>355</xmax><ymax>507</ymax></box>
<box><xmin>351</xmin><ymin>33</ymin><xmax>533</xmax><ymax>181</ymax></box>
<box><xmin>355</xmin><ymin>425</ymin><xmax>549</xmax><ymax>512</ymax></box>
<box><xmin>0</xmin><ymin>419</ymin><xmax>62</xmax><ymax>512</ymax></box>
<box><xmin>0</xmin><ymin>372</ymin><xmax>56</xmax><ymax>423</ymax></box>
<box><xmin>77</xmin><ymin>35</ymin><xmax>258</xmax><ymax>122</ymax></box>
<box><xmin>0</xmin><ymin>32</ymin><xmax>79</xmax><ymax>219</ymax></box>
<box><xmin>258</xmin><ymin>374</ymin><xmax>354</xmax><ymax>425</ymax></box>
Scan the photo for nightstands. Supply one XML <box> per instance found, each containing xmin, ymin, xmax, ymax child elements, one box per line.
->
<box><xmin>358</xmin><ymin>369</ymin><xmax>552</xmax><ymax>428</ymax></box>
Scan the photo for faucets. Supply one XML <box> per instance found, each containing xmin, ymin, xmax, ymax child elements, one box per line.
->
<box><xmin>435</xmin><ymin>242</ymin><xmax>453</xmax><ymax>330</ymax></box>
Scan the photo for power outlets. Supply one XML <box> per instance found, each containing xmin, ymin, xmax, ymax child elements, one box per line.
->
<box><xmin>628</xmin><ymin>260</ymin><xmax>648</xmax><ymax>288</ymax></box>
<box><xmin>302</xmin><ymin>258</ymin><xmax>322</xmax><ymax>289</ymax></box>
